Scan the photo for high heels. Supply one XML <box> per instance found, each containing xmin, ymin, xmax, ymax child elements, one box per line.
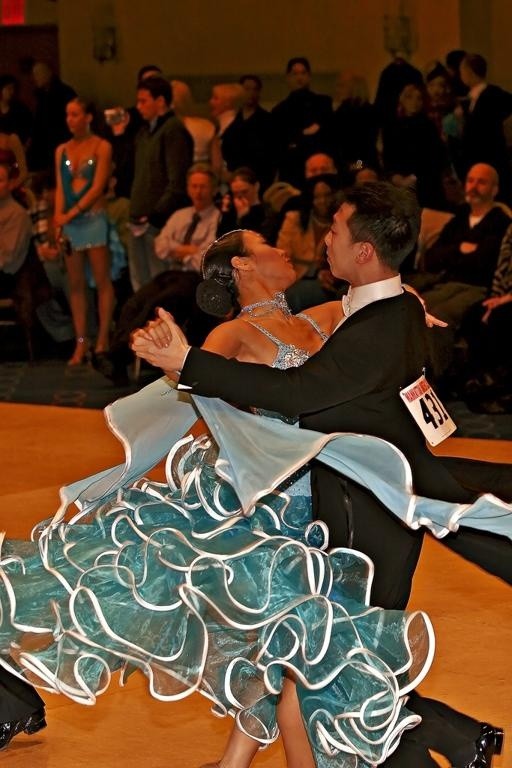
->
<box><xmin>67</xmin><ymin>337</ymin><xmax>92</xmax><ymax>366</ymax></box>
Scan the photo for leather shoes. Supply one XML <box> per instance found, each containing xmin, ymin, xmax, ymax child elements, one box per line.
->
<box><xmin>0</xmin><ymin>706</ymin><xmax>48</xmax><ymax>750</ymax></box>
<box><xmin>470</xmin><ymin>720</ymin><xmax>503</xmax><ymax>768</ymax></box>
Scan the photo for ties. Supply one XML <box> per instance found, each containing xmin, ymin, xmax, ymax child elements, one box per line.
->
<box><xmin>179</xmin><ymin>213</ymin><xmax>202</xmax><ymax>268</ymax></box>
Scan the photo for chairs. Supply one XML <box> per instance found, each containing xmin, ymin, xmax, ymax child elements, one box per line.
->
<box><xmin>0</xmin><ymin>186</ymin><xmax>37</xmax><ymax>361</ymax></box>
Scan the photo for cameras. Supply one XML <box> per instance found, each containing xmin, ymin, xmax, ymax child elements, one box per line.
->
<box><xmin>104</xmin><ymin>109</ymin><xmax>125</xmax><ymax>125</ymax></box>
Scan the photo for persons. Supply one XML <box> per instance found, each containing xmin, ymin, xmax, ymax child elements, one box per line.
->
<box><xmin>128</xmin><ymin>179</ymin><xmax>506</xmax><ymax>767</ymax></box>
<box><xmin>130</xmin><ymin>230</ymin><xmax>449</xmax><ymax>767</ymax></box>
<box><xmin>0</xmin><ymin>51</ymin><xmax>512</xmax><ymax>413</ymax></box>
<box><xmin>1</xmin><ymin>652</ymin><xmax>46</xmax><ymax>750</ymax></box>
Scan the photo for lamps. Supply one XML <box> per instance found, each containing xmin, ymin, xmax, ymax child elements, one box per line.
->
<box><xmin>384</xmin><ymin>13</ymin><xmax>412</xmax><ymax>58</ymax></box>
<box><xmin>92</xmin><ymin>25</ymin><xmax>116</xmax><ymax>64</ymax></box>
